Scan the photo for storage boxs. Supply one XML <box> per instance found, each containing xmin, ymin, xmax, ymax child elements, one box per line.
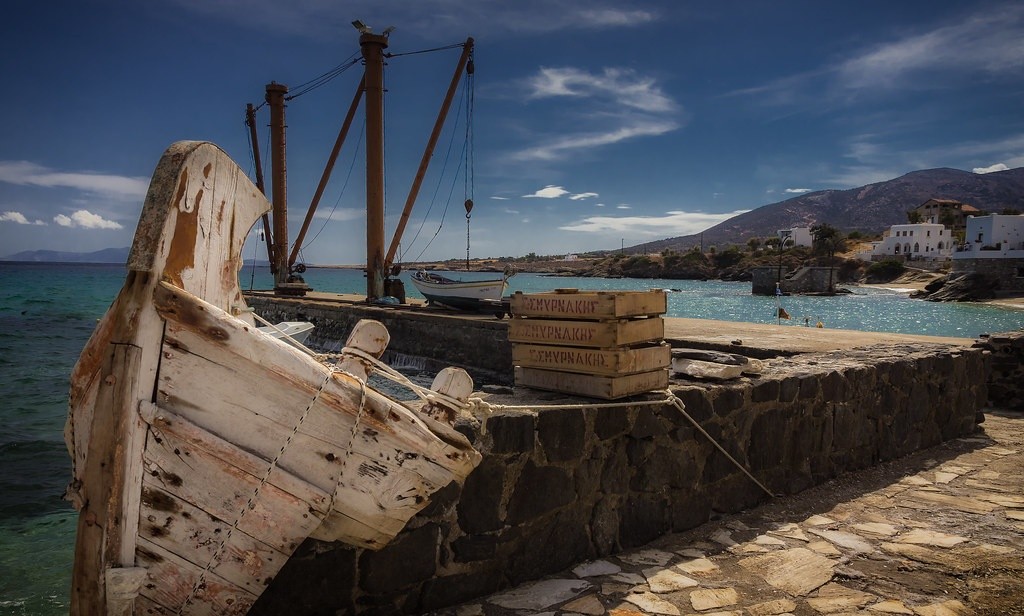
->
<box><xmin>504</xmin><ymin>288</ymin><xmax>672</xmax><ymax>399</ymax></box>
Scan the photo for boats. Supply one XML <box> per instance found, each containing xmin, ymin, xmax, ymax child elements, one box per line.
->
<box><xmin>409</xmin><ymin>262</ymin><xmax>519</xmax><ymax>312</ymax></box>
